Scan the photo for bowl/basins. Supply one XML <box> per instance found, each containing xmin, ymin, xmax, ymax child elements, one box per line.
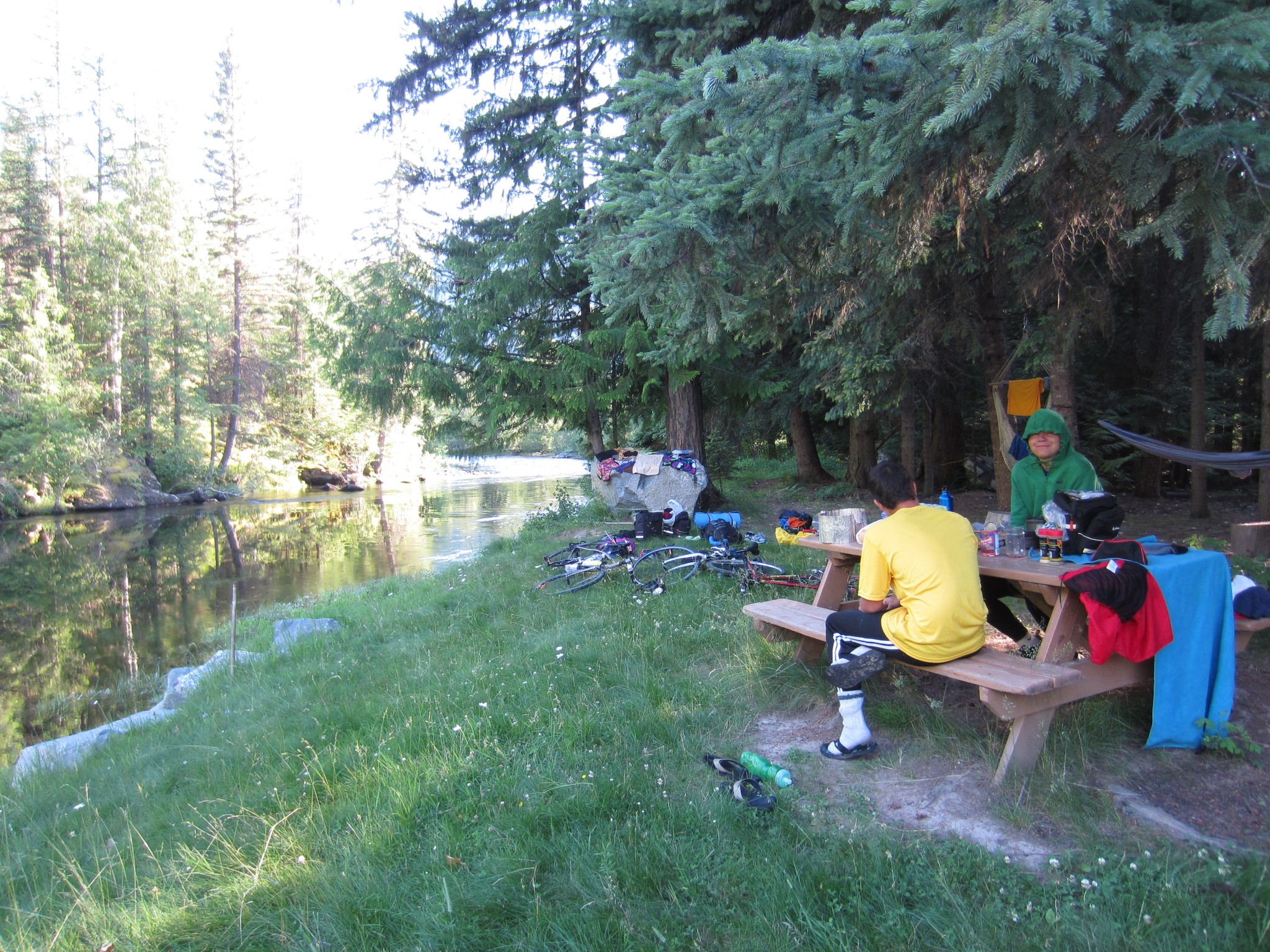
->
<box><xmin>1023</xmin><ymin>531</ymin><xmax>1039</xmax><ymax>550</ymax></box>
<box><xmin>1026</xmin><ymin>517</ymin><xmax>1046</xmax><ymax>531</ymax></box>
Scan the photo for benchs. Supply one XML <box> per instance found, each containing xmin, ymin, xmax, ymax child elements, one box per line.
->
<box><xmin>743</xmin><ymin>599</ymin><xmax>1086</xmax><ymax>696</ymax></box>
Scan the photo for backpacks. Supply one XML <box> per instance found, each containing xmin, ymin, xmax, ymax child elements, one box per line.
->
<box><xmin>694</xmin><ymin>510</ymin><xmax>742</xmax><ymax>544</ymax></box>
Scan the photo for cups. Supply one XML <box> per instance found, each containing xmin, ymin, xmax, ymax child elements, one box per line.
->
<box><xmin>1006</xmin><ymin>527</ymin><xmax>1026</xmax><ymax>557</ymax></box>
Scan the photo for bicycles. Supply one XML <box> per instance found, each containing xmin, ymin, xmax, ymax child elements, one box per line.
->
<box><xmin>534</xmin><ymin>531</ymin><xmax>894</xmax><ymax>603</ymax></box>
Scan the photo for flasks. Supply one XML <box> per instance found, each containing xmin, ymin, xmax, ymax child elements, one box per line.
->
<box><xmin>939</xmin><ymin>485</ymin><xmax>953</xmax><ymax>512</ymax></box>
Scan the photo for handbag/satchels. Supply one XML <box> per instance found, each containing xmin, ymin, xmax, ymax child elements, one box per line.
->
<box><xmin>662</xmin><ymin>499</ymin><xmax>691</xmax><ymax>538</ymax></box>
<box><xmin>633</xmin><ymin>510</ymin><xmax>664</xmax><ymax>541</ymax></box>
<box><xmin>1053</xmin><ymin>488</ymin><xmax>1125</xmax><ymax>554</ymax></box>
<box><xmin>779</xmin><ymin>510</ymin><xmax>813</xmax><ymax>535</ymax></box>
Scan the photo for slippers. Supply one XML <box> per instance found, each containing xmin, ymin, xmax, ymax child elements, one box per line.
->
<box><xmin>823</xmin><ymin>649</ymin><xmax>887</xmax><ymax>689</ymax></box>
<box><xmin>820</xmin><ymin>738</ymin><xmax>877</xmax><ymax>759</ymax></box>
<box><xmin>719</xmin><ymin>778</ymin><xmax>777</xmax><ymax>809</ymax></box>
<box><xmin>702</xmin><ymin>755</ymin><xmax>763</xmax><ymax>784</ymax></box>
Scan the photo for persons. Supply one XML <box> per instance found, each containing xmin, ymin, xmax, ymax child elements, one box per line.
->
<box><xmin>819</xmin><ymin>461</ymin><xmax>989</xmax><ymax>760</ymax></box>
<box><xmin>980</xmin><ymin>409</ymin><xmax>1104</xmax><ymax>659</ymax></box>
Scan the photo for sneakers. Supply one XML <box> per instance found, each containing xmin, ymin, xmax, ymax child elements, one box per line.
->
<box><xmin>751</xmin><ymin>534</ymin><xmax>765</xmax><ymax>543</ymax></box>
<box><xmin>745</xmin><ymin>531</ymin><xmax>756</xmax><ymax>541</ymax></box>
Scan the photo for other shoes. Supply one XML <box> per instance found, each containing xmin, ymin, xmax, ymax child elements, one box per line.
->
<box><xmin>1014</xmin><ymin>634</ymin><xmax>1042</xmax><ymax>658</ymax></box>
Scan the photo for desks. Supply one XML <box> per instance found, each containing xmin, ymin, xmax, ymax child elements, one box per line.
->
<box><xmin>792</xmin><ymin>524</ymin><xmax>1236</xmax><ymax>787</ymax></box>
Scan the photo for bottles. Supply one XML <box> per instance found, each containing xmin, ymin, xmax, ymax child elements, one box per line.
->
<box><xmin>672</xmin><ymin>450</ymin><xmax>683</xmax><ymax>454</ymax></box>
<box><xmin>583</xmin><ymin>560</ymin><xmax>607</xmax><ymax>566</ymax></box>
<box><xmin>584</xmin><ymin>553</ymin><xmax>605</xmax><ymax>561</ymax></box>
<box><xmin>1038</xmin><ymin>528</ymin><xmax>1064</xmax><ymax>565</ymax></box>
<box><xmin>565</xmin><ymin>564</ymin><xmax>582</xmax><ymax>572</ymax></box>
<box><xmin>740</xmin><ymin>751</ymin><xmax>792</xmax><ymax>788</ymax></box>
